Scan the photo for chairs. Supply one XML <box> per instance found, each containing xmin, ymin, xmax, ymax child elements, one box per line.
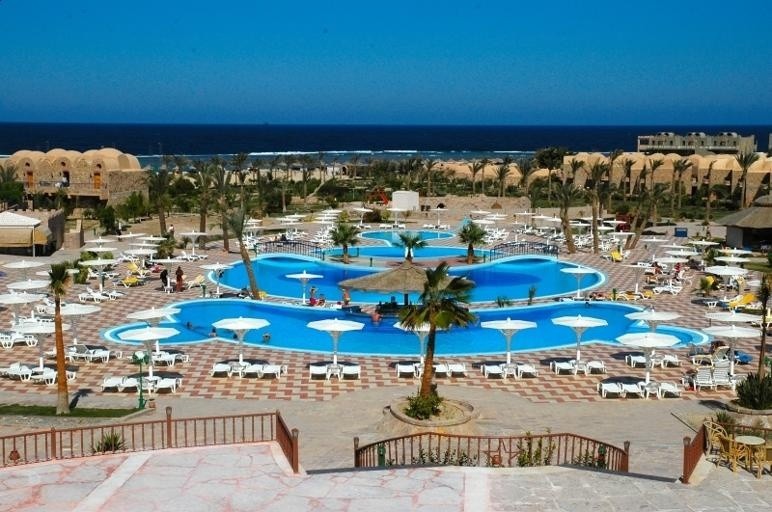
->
<box><xmin>234</xmin><ymin>223</ymin><xmax>633</xmax><ymax>261</ymax></box>
<box><xmin>294</xmin><ymin>299</ymin><xmax>345</xmax><ymax>311</ymax></box>
<box><xmin>557</xmin><ymin>290</ymin><xmax>650</xmax><ymax>303</ymax></box>
<box><xmin>636</xmin><ymin>259</ymin><xmax>772</xmax><ymax>333</ymax></box>
<box><xmin>237</xmin><ymin>287</ymin><xmax>265</xmax><ymax>303</ymax></box>
<box><xmin>1</xmin><ymin>252</ymin><xmax>209</xmax><ymax>350</ymax></box>
<box><xmin>0</xmin><ymin>345</ymin><xmax>746</xmax><ymax>399</ymax></box>
<box><xmin>702</xmin><ymin>421</ymin><xmax>772</xmax><ymax>479</ymax></box>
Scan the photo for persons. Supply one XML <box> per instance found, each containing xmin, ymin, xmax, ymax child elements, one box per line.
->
<box><xmin>168</xmin><ymin>223</ymin><xmax>175</xmax><ymax>234</ymax></box>
<box><xmin>371</xmin><ymin>310</ymin><xmax>383</xmax><ymax>322</ymax></box>
<box><xmin>308</xmin><ymin>284</ymin><xmax>352</xmax><ymax>310</ymax></box>
<box><xmin>695</xmin><ymin>225</ymin><xmax>712</xmax><ymax>241</ymax></box>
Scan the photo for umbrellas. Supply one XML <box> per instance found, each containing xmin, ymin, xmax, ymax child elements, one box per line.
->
<box><xmin>623</xmin><ymin>308</ymin><xmax>682</xmax><ymax>333</ymax></box>
<box><xmin>614</xmin><ymin>330</ymin><xmax>681</xmax><ymax>381</ymax></box>
<box><xmin>560</xmin><ymin>265</ymin><xmax>598</xmax><ymax>298</ymax></box>
<box><xmin>704</xmin><ymin>311</ymin><xmax>763</xmax><ymax>324</ymax></box>
<box><xmin>0</xmin><ymin>229</ymin><xmax>218</xmax><ymax>348</ymax></box>
<box><xmin>550</xmin><ymin>314</ymin><xmax>610</xmax><ymax>360</ymax></box>
<box><xmin>620</xmin><ymin>233</ymin><xmax>754</xmax><ymax>304</ymax></box>
<box><xmin>700</xmin><ymin>325</ymin><xmax>763</xmax><ymax>373</ymax></box>
<box><xmin>210</xmin><ymin>313</ymin><xmax>273</xmax><ymax>363</ymax></box>
<box><xmin>284</xmin><ymin>270</ymin><xmax>324</xmax><ymax>305</ymax></box>
<box><xmin>125</xmin><ymin>305</ymin><xmax>184</xmax><ymax>355</ymax></box>
<box><xmin>7</xmin><ymin>320</ymin><xmax>72</xmax><ymax>370</ymax></box>
<box><xmin>116</xmin><ymin>325</ymin><xmax>181</xmax><ymax>380</ymax></box>
<box><xmin>478</xmin><ymin>316</ymin><xmax>537</xmax><ymax>363</ymax></box>
<box><xmin>392</xmin><ymin>319</ymin><xmax>451</xmax><ymax>362</ymax></box>
<box><xmin>305</xmin><ymin>315</ymin><xmax>367</xmax><ymax>362</ymax></box>
